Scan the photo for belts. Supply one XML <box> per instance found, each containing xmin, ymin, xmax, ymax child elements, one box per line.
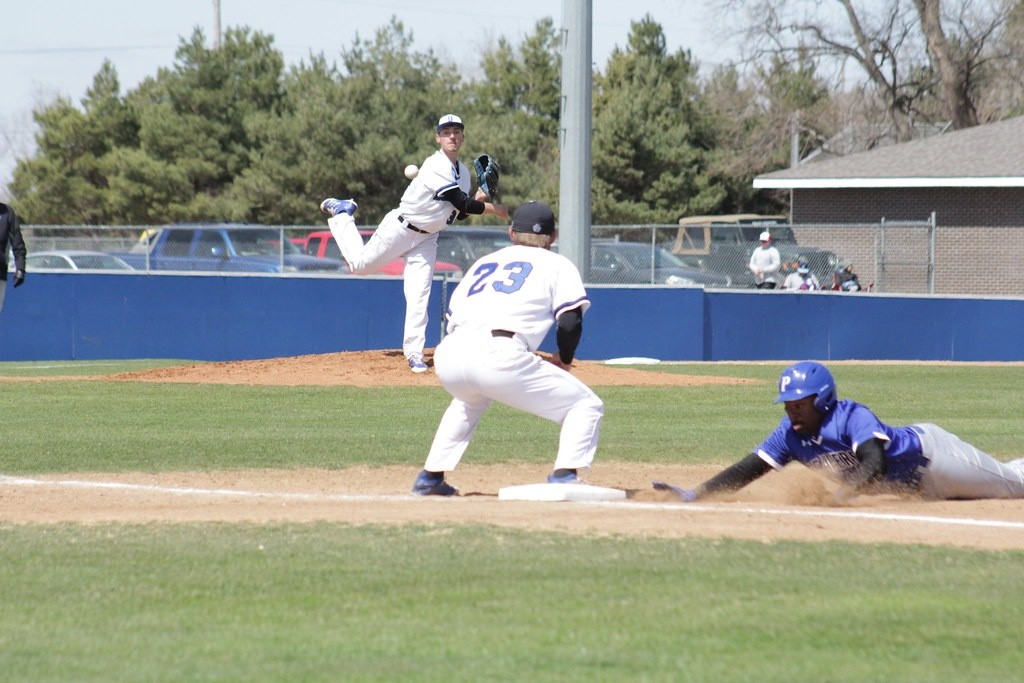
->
<box><xmin>491</xmin><ymin>329</ymin><xmax>514</xmax><ymax>338</ymax></box>
<box><xmin>910</xmin><ymin>425</ymin><xmax>929</xmax><ymax>484</ymax></box>
<box><xmin>397</xmin><ymin>215</ymin><xmax>430</xmax><ymax>234</ymax></box>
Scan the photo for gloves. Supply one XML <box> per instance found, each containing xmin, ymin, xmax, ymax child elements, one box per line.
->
<box><xmin>12</xmin><ymin>270</ymin><xmax>25</xmax><ymax>288</ymax></box>
<box><xmin>653</xmin><ymin>480</ymin><xmax>696</xmax><ymax>503</ymax></box>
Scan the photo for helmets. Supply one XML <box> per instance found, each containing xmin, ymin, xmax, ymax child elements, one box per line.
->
<box><xmin>773</xmin><ymin>361</ymin><xmax>836</xmax><ymax>416</ymax></box>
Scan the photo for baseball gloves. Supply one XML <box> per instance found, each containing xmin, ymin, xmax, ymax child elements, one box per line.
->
<box><xmin>473</xmin><ymin>153</ymin><xmax>500</xmax><ymax>198</ymax></box>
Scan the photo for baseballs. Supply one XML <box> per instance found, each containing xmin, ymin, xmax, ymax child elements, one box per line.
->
<box><xmin>404</xmin><ymin>165</ymin><xmax>419</xmax><ymax>180</ymax></box>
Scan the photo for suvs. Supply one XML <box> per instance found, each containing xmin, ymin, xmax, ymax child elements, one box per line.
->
<box><xmin>670</xmin><ymin>210</ymin><xmax>844</xmax><ymax>290</ymax></box>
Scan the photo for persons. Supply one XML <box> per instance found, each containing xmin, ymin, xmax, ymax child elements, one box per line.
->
<box><xmin>653</xmin><ymin>362</ymin><xmax>1024</xmax><ymax>504</ymax></box>
<box><xmin>320</xmin><ymin>113</ymin><xmax>509</xmax><ymax>372</ymax></box>
<box><xmin>781</xmin><ymin>260</ymin><xmax>827</xmax><ymax>289</ymax></box>
<box><xmin>412</xmin><ymin>200</ymin><xmax>604</xmax><ymax>496</ymax></box>
<box><xmin>749</xmin><ymin>230</ymin><xmax>782</xmax><ymax>289</ymax></box>
<box><xmin>0</xmin><ymin>203</ymin><xmax>27</xmax><ymax>314</ymax></box>
<box><xmin>829</xmin><ymin>263</ymin><xmax>874</xmax><ymax>292</ymax></box>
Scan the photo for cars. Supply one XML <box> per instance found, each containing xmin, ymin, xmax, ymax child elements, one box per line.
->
<box><xmin>8</xmin><ymin>249</ymin><xmax>136</xmax><ymax>272</ymax></box>
<box><xmin>548</xmin><ymin>240</ymin><xmax>732</xmax><ymax>288</ymax></box>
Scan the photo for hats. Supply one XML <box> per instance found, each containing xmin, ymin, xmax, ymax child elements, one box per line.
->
<box><xmin>798</xmin><ymin>262</ymin><xmax>810</xmax><ymax>273</ymax></box>
<box><xmin>841</xmin><ymin>258</ymin><xmax>854</xmax><ymax>270</ymax></box>
<box><xmin>437</xmin><ymin>113</ymin><xmax>465</xmax><ymax>132</ymax></box>
<box><xmin>759</xmin><ymin>231</ymin><xmax>773</xmax><ymax>241</ymax></box>
<box><xmin>511</xmin><ymin>201</ymin><xmax>555</xmax><ymax>234</ymax></box>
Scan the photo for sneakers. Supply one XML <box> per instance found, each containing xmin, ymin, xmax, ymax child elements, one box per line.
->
<box><xmin>320</xmin><ymin>198</ymin><xmax>360</xmax><ymax>217</ymax></box>
<box><xmin>548</xmin><ymin>474</ymin><xmax>591</xmax><ymax>486</ymax></box>
<box><xmin>411</xmin><ymin>471</ymin><xmax>459</xmax><ymax>496</ymax></box>
<box><xmin>408</xmin><ymin>355</ymin><xmax>428</xmax><ymax>373</ymax></box>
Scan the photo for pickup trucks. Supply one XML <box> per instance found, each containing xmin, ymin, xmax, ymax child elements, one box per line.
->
<box><xmin>104</xmin><ymin>223</ymin><xmax>516</xmax><ymax>282</ymax></box>
